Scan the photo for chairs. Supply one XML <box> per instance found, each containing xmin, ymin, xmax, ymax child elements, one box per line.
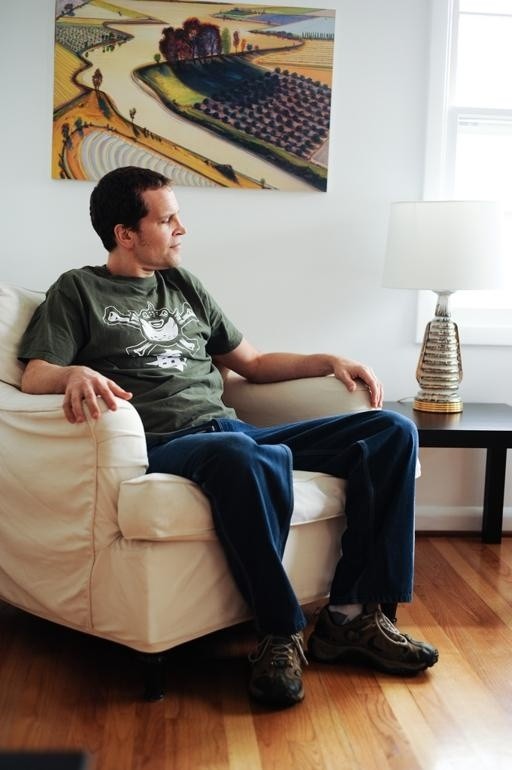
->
<box><xmin>1</xmin><ymin>284</ymin><xmax>423</xmax><ymax>699</ymax></box>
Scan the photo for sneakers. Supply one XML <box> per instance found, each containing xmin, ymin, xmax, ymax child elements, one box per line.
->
<box><xmin>306</xmin><ymin>601</ymin><xmax>441</xmax><ymax>675</ymax></box>
<box><xmin>247</xmin><ymin>627</ymin><xmax>307</xmax><ymax>712</ymax></box>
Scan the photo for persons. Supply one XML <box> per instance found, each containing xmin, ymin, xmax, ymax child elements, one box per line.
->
<box><xmin>13</xmin><ymin>165</ymin><xmax>441</xmax><ymax>710</ymax></box>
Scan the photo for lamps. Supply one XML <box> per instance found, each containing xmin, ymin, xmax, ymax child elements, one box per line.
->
<box><xmin>383</xmin><ymin>197</ymin><xmax>494</xmax><ymax>413</ymax></box>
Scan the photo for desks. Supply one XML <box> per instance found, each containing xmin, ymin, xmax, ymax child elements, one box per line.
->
<box><xmin>380</xmin><ymin>400</ymin><xmax>511</xmax><ymax>544</ymax></box>
